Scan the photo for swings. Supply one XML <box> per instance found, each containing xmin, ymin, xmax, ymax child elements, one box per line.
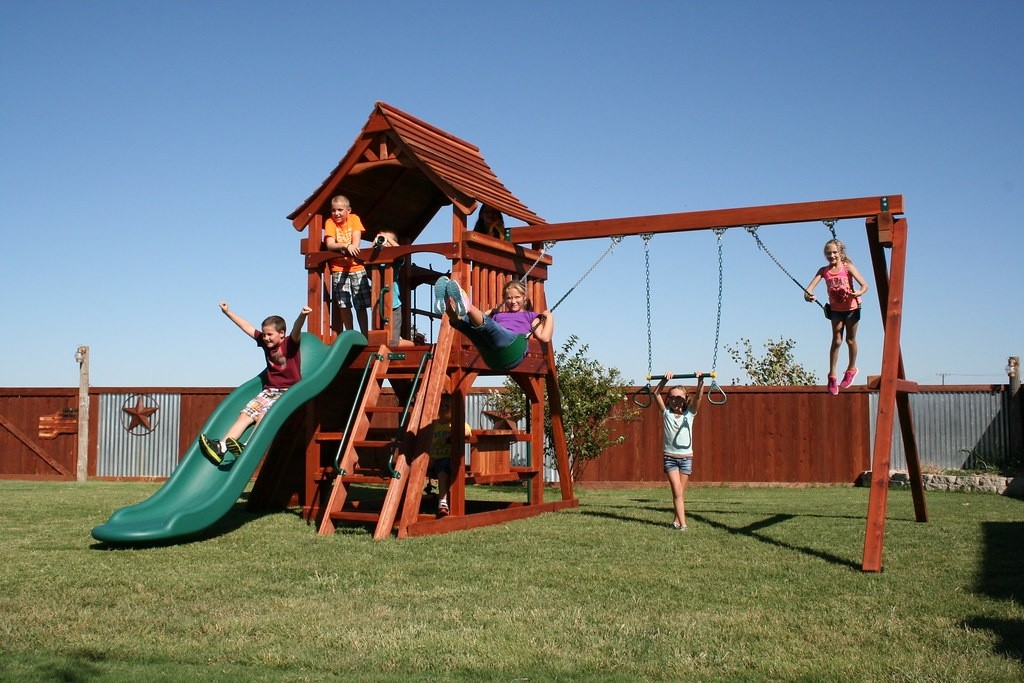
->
<box><xmin>469</xmin><ymin>234</ymin><xmax>624</xmax><ymax>370</ymax></box>
<box><xmin>744</xmin><ymin>222</ymin><xmax>863</xmax><ymax>322</ymax></box>
<box><xmin>640</xmin><ymin>226</ymin><xmax>719</xmax><ymax>381</ymax></box>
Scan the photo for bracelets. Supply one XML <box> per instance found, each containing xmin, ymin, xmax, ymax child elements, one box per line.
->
<box><xmin>695</xmin><ymin>395</ymin><xmax>702</xmax><ymax>399</ymax></box>
<box><xmin>347</xmin><ymin>244</ymin><xmax>350</xmax><ymax>248</ymax></box>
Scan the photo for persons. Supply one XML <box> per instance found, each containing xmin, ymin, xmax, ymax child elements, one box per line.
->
<box><xmin>426</xmin><ymin>393</ymin><xmax>472</xmax><ymax>516</ymax></box>
<box><xmin>200</xmin><ymin>300</ymin><xmax>313</xmax><ymax>464</ymax></box>
<box><xmin>805</xmin><ymin>240</ymin><xmax>868</xmax><ymax>396</ymax></box>
<box><xmin>653</xmin><ymin>371</ymin><xmax>704</xmax><ymax>531</ymax></box>
<box><xmin>433</xmin><ymin>276</ymin><xmax>554</xmax><ymax>369</ymax></box>
<box><xmin>324</xmin><ymin>194</ymin><xmax>426</xmax><ymax>348</ymax></box>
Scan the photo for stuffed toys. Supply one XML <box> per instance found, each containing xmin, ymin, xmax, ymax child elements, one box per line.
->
<box><xmin>1006</xmin><ymin>358</ymin><xmax>1018</xmax><ymax>377</ymax></box>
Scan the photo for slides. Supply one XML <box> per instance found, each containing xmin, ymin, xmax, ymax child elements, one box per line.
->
<box><xmin>89</xmin><ymin>329</ymin><xmax>368</xmax><ymax>544</ymax></box>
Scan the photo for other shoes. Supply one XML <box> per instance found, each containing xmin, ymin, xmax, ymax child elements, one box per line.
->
<box><xmin>447</xmin><ymin>279</ymin><xmax>470</xmax><ymax>315</ymax></box>
<box><xmin>200</xmin><ymin>433</ymin><xmax>225</xmax><ymax>464</ymax></box>
<box><xmin>671</xmin><ymin>522</ymin><xmax>680</xmax><ymax>528</ymax></box>
<box><xmin>438</xmin><ymin>499</ymin><xmax>449</xmax><ymax>515</ymax></box>
<box><xmin>434</xmin><ymin>276</ymin><xmax>449</xmax><ymax>314</ymax></box>
<box><xmin>680</xmin><ymin>525</ymin><xmax>688</xmax><ymax>531</ymax></box>
<box><xmin>226</xmin><ymin>437</ymin><xmax>245</xmax><ymax>460</ymax></box>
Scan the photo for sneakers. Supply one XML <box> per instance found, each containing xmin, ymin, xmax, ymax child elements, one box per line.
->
<box><xmin>840</xmin><ymin>367</ymin><xmax>858</xmax><ymax>388</ymax></box>
<box><xmin>827</xmin><ymin>372</ymin><xmax>839</xmax><ymax>396</ymax></box>
